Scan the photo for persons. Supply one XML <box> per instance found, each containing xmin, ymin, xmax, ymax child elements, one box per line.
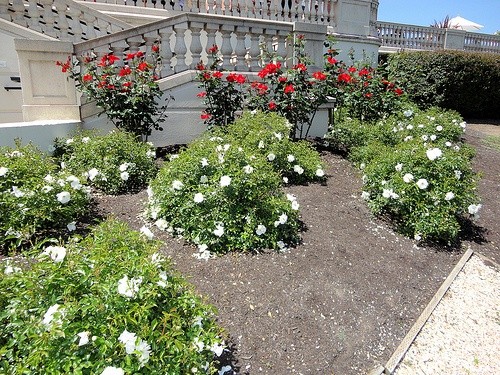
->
<box><xmin>125</xmin><ymin>0</ymin><xmax>330</xmax><ymax>25</ymax></box>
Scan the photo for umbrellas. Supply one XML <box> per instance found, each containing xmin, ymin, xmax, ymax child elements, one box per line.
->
<box><xmin>426</xmin><ymin>16</ymin><xmax>484</xmax><ymax>38</ymax></box>
